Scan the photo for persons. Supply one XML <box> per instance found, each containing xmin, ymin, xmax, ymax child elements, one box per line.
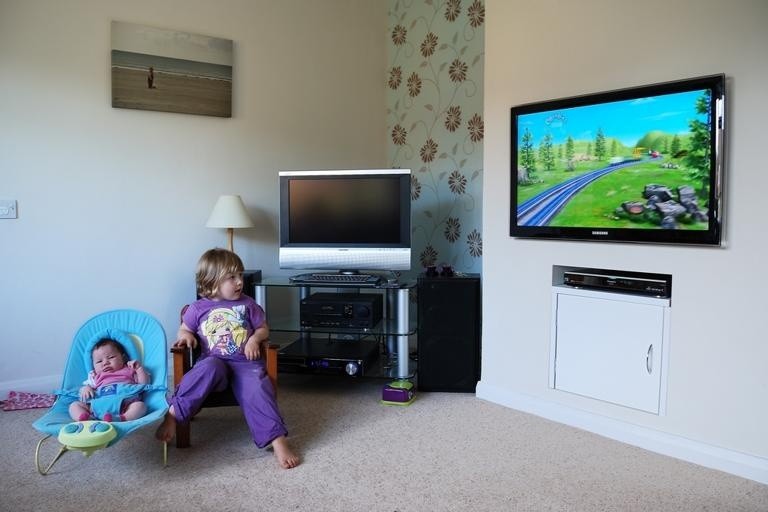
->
<box><xmin>147</xmin><ymin>67</ymin><xmax>154</xmax><ymax>89</ymax></box>
<box><xmin>68</xmin><ymin>339</ymin><xmax>150</xmax><ymax>423</ymax></box>
<box><xmin>155</xmin><ymin>248</ymin><xmax>300</xmax><ymax>470</ymax></box>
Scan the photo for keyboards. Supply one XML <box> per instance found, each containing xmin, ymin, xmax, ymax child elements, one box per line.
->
<box><xmin>289</xmin><ymin>272</ymin><xmax>382</xmax><ymax>287</ymax></box>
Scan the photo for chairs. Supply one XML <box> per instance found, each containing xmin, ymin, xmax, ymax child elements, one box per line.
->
<box><xmin>171</xmin><ymin>303</ymin><xmax>281</xmax><ymax>449</ymax></box>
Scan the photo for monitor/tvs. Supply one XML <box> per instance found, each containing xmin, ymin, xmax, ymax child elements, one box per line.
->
<box><xmin>278</xmin><ymin>167</ymin><xmax>412</xmax><ymax>277</ymax></box>
<box><xmin>509</xmin><ymin>71</ymin><xmax>727</xmax><ymax>246</ymax></box>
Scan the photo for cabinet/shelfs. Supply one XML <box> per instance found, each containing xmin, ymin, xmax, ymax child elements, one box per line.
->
<box><xmin>553</xmin><ymin>284</ymin><xmax>669</xmax><ymax>416</ymax></box>
<box><xmin>254</xmin><ymin>273</ymin><xmax>419</xmax><ymax>386</ymax></box>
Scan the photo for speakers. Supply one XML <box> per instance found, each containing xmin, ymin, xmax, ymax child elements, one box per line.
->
<box><xmin>195</xmin><ymin>269</ymin><xmax>262</xmax><ymax>300</ymax></box>
<box><xmin>416</xmin><ymin>271</ymin><xmax>481</xmax><ymax>394</ymax></box>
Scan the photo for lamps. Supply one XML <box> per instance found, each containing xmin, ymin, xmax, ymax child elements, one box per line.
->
<box><xmin>204</xmin><ymin>193</ymin><xmax>258</xmax><ymax>254</ymax></box>
